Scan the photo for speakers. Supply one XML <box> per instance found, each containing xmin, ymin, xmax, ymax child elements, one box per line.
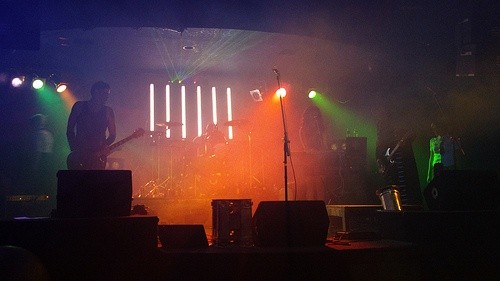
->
<box><xmin>250</xmin><ymin>200</ymin><xmax>331</xmax><ymax>249</ymax></box>
<box><xmin>158</xmin><ymin>224</ymin><xmax>209</xmax><ymax>250</ymax></box>
<box><xmin>211</xmin><ymin>198</ymin><xmax>253</xmax><ymax>249</ymax></box>
<box><xmin>55</xmin><ymin>169</ymin><xmax>132</xmax><ymax>218</ymax></box>
<box><xmin>345</xmin><ymin>137</ymin><xmax>368</xmax><ymax>205</ymax></box>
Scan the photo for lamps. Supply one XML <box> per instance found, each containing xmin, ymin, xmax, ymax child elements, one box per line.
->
<box><xmin>32</xmin><ymin>73</ymin><xmax>44</xmax><ymax>90</ymax></box>
<box><xmin>248</xmin><ymin>86</ymin><xmax>265</xmax><ymax>103</ymax></box>
<box><xmin>48</xmin><ymin>71</ymin><xmax>67</xmax><ymax>92</ymax></box>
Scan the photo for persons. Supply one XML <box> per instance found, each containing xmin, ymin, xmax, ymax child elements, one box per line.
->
<box><xmin>427</xmin><ymin>118</ymin><xmax>462</xmax><ymax>203</ymax></box>
<box><xmin>376</xmin><ymin>118</ymin><xmax>419</xmax><ymax>205</ymax></box>
<box><xmin>205</xmin><ymin>122</ymin><xmax>227</xmax><ymax>158</ymax></box>
<box><xmin>30</xmin><ymin>114</ymin><xmax>54</xmax><ymax>204</ymax></box>
<box><xmin>66</xmin><ymin>81</ymin><xmax>116</xmax><ymax>171</ymax></box>
<box><xmin>300</xmin><ymin>88</ymin><xmax>332</xmax><ymax>204</ymax></box>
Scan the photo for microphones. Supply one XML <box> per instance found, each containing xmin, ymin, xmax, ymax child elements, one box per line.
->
<box><xmin>273</xmin><ymin>68</ymin><xmax>280</xmax><ymax>77</ymax></box>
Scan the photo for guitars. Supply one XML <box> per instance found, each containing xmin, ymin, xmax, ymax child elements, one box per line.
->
<box><xmin>376</xmin><ymin>127</ymin><xmax>416</xmax><ymax>176</ymax></box>
<box><xmin>66</xmin><ymin>128</ymin><xmax>146</xmax><ymax>171</ymax></box>
